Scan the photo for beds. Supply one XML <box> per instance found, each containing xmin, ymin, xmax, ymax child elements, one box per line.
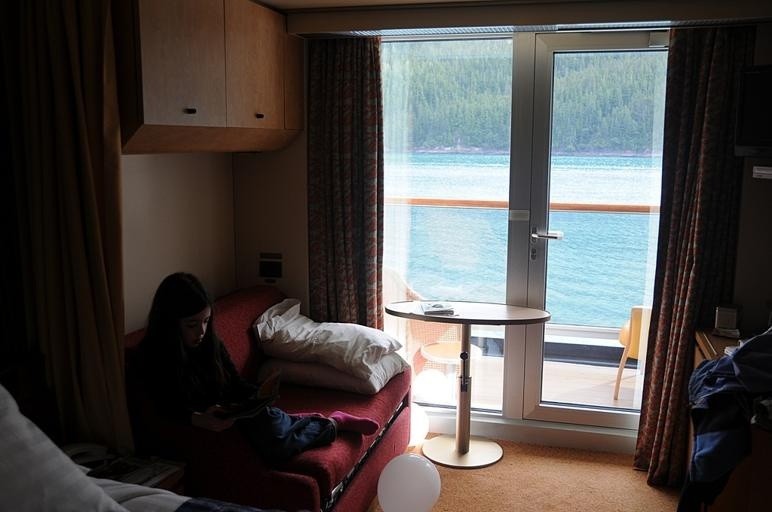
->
<box><xmin>75</xmin><ymin>463</ymin><xmax>288</xmax><ymax>512</ymax></box>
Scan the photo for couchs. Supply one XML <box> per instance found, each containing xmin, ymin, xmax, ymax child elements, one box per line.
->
<box><xmin>125</xmin><ymin>285</ymin><xmax>411</xmax><ymax>512</ymax></box>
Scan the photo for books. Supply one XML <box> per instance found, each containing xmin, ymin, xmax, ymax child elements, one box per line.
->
<box><xmin>421</xmin><ymin>302</ymin><xmax>454</xmax><ymax>316</ymax></box>
<box><xmin>210</xmin><ymin>369</ymin><xmax>283</xmax><ymax>420</ymax></box>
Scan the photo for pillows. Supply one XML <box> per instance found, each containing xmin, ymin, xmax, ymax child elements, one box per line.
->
<box><xmin>252</xmin><ymin>298</ymin><xmax>402</xmax><ymax>381</ymax></box>
<box><xmin>0</xmin><ymin>384</ymin><xmax>139</xmax><ymax>512</ymax></box>
<box><xmin>258</xmin><ymin>350</ymin><xmax>411</xmax><ymax>395</ymax></box>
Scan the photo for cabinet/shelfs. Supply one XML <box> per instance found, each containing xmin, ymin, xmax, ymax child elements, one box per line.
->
<box><xmin>111</xmin><ymin>0</ymin><xmax>305</xmax><ymax>155</ymax></box>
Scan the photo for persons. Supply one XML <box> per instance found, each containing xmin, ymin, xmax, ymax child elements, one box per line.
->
<box><xmin>122</xmin><ymin>272</ymin><xmax>379</xmax><ymax>477</ymax></box>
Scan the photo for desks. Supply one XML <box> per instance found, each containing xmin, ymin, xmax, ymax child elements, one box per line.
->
<box><xmin>420</xmin><ymin>340</ymin><xmax>482</xmax><ymax>378</ymax></box>
<box><xmin>685</xmin><ymin>327</ymin><xmax>772</xmax><ymax>512</ymax></box>
<box><xmin>385</xmin><ymin>301</ymin><xmax>552</xmax><ymax>470</ymax></box>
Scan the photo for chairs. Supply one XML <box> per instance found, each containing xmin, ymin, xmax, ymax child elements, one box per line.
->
<box><xmin>382</xmin><ymin>267</ymin><xmax>462</xmax><ymax>381</ymax></box>
<box><xmin>612</xmin><ymin>306</ymin><xmax>643</xmax><ymax>400</ymax></box>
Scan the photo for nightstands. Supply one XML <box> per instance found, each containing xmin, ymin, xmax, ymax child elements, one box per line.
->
<box><xmin>87</xmin><ymin>456</ymin><xmax>187</xmax><ymax>496</ymax></box>
